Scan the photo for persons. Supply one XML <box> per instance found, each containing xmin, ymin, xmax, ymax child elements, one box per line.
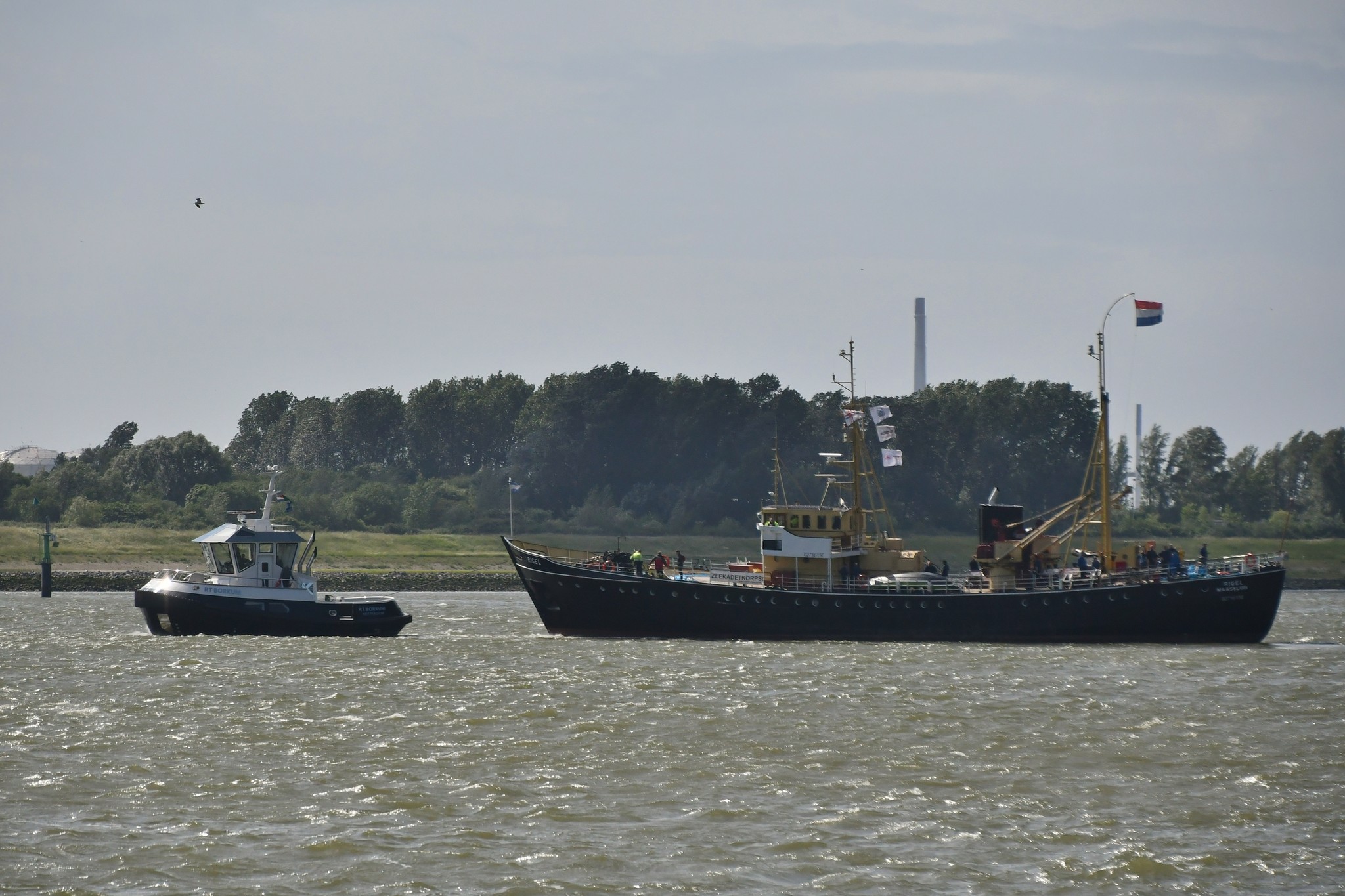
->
<box><xmin>942</xmin><ymin>560</ymin><xmax>950</xmax><ymax>576</ymax></box>
<box><xmin>1078</xmin><ymin>551</ymin><xmax>1088</xmax><ymax>578</ymax></box>
<box><xmin>241</xmin><ymin>554</ymin><xmax>250</xmax><ymax>561</ymax></box>
<box><xmin>776</xmin><ymin>515</ymin><xmax>841</xmax><ymax>529</ymax></box>
<box><xmin>1158</xmin><ymin>543</ymin><xmax>1179</xmax><ymax>568</ymax></box>
<box><xmin>1147</xmin><ymin>545</ymin><xmax>1159</xmax><ymax>569</ymax></box>
<box><xmin>276</xmin><ymin>556</ymin><xmax>282</xmax><ymax>567</ymax></box>
<box><xmin>1141</xmin><ymin>546</ymin><xmax>1150</xmax><ymax>569</ymax></box>
<box><xmin>926</xmin><ymin>561</ymin><xmax>937</xmax><ymax>573</ymax></box>
<box><xmin>649</xmin><ymin>551</ymin><xmax>668</xmax><ymax>578</ymax></box>
<box><xmin>1200</xmin><ymin>543</ymin><xmax>1210</xmax><ymax>564</ymax></box>
<box><xmin>676</xmin><ymin>550</ymin><xmax>686</xmax><ymax>580</ymax></box>
<box><xmin>630</xmin><ymin>550</ymin><xmax>643</xmax><ymax>575</ymax></box>
<box><xmin>1092</xmin><ymin>556</ymin><xmax>1101</xmax><ymax>574</ymax></box>
<box><xmin>765</xmin><ymin>518</ymin><xmax>779</xmax><ymax>527</ymax></box>
<box><xmin>280</xmin><ymin>564</ymin><xmax>294</xmax><ymax>588</ymax></box>
<box><xmin>970</xmin><ymin>555</ymin><xmax>982</xmax><ymax>572</ymax></box>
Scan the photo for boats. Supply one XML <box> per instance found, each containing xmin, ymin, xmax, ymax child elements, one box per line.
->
<box><xmin>133</xmin><ymin>461</ymin><xmax>414</xmax><ymax>637</ymax></box>
<box><xmin>500</xmin><ymin>288</ymin><xmax>1286</xmax><ymax>649</ymax></box>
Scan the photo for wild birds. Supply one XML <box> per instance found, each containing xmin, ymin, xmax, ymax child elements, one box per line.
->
<box><xmin>193</xmin><ymin>197</ymin><xmax>206</xmax><ymax>210</ymax></box>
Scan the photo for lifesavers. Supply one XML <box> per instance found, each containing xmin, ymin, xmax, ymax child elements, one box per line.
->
<box><xmin>857</xmin><ymin>575</ymin><xmax>869</xmax><ymax>588</ymax></box>
<box><xmin>1246</xmin><ymin>553</ymin><xmax>1256</xmax><ymax>566</ymax></box>
<box><xmin>276</xmin><ymin>580</ymin><xmax>281</xmax><ymax>588</ymax></box>
<box><xmin>602</xmin><ymin>561</ymin><xmax>616</xmax><ymax>572</ymax></box>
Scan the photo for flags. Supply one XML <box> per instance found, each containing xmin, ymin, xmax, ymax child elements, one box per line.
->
<box><xmin>1134</xmin><ymin>300</ymin><xmax>1163</xmax><ymax>327</ymax></box>
<box><xmin>876</xmin><ymin>424</ymin><xmax>896</xmax><ymax>442</ymax></box>
<box><xmin>510</xmin><ymin>482</ymin><xmax>523</xmax><ymax>495</ymax></box>
<box><xmin>842</xmin><ymin>408</ymin><xmax>865</xmax><ymax>426</ymax></box>
<box><xmin>881</xmin><ymin>448</ymin><xmax>903</xmax><ymax>467</ymax></box>
<box><xmin>869</xmin><ymin>404</ymin><xmax>892</xmax><ymax>424</ymax></box>
